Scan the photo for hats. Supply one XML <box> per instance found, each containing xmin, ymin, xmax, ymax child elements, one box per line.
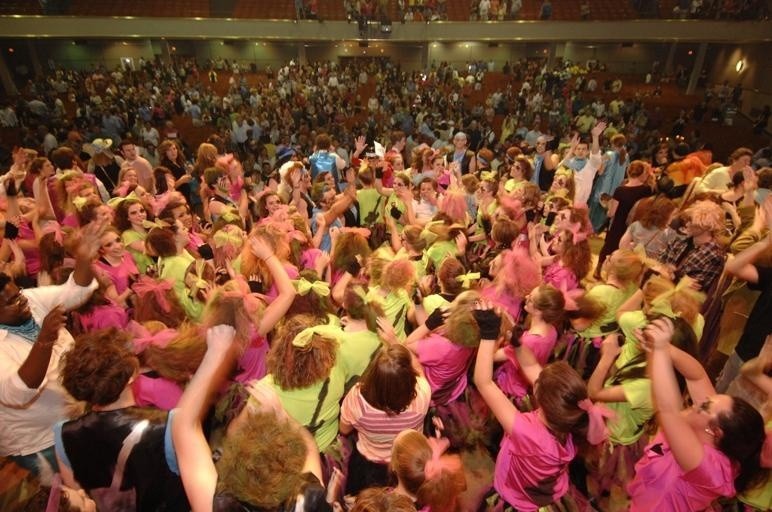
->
<box><xmin>80</xmin><ymin>138</ymin><xmax>114</xmax><ymax>154</ymax></box>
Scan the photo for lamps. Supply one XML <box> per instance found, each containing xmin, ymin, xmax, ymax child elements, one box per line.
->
<box><xmin>735</xmin><ymin>60</ymin><xmax>743</xmax><ymax>73</ymax></box>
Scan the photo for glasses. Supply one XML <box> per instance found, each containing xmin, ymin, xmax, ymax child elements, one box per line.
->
<box><xmin>0</xmin><ymin>287</ymin><xmax>24</xmax><ymax>308</ymax></box>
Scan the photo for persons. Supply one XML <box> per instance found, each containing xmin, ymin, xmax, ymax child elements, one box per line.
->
<box><xmin>671</xmin><ymin>0</ymin><xmax>772</xmax><ymax>21</ymax></box>
<box><xmin>579</xmin><ymin>0</ymin><xmax>592</xmax><ymax>19</ymax></box>
<box><xmin>293</xmin><ymin>0</ymin><xmax>525</xmax><ymax>23</ymax></box>
<box><xmin>0</xmin><ymin>49</ymin><xmax>772</xmax><ymax>512</ymax></box>
<box><xmin>654</xmin><ymin>2</ymin><xmax>663</xmax><ymax>19</ymax></box>
<box><xmin>536</xmin><ymin>0</ymin><xmax>553</xmax><ymax>20</ymax></box>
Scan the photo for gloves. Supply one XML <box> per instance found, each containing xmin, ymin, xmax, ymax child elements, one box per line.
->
<box><xmin>346</xmin><ymin>256</ymin><xmax>362</xmax><ymax>278</ymax></box>
<box><xmin>247</xmin><ymin>279</ymin><xmax>264</xmax><ymax>294</ymax></box>
<box><xmin>544</xmin><ymin>211</ymin><xmax>559</xmax><ymax>227</ymax></box>
<box><xmin>195</xmin><ymin>243</ymin><xmax>215</xmax><ymax>261</ymax></box>
<box><xmin>537</xmin><ymin>202</ymin><xmax>549</xmax><ymax>220</ymax></box>
<box><xmin>479</xmin><ymin>213</ymin><xmax>494</xmax><ymax>235</ymax></box>
<box><xmin>389</xmin><ymin>206</ymin><xmax>402</xmax><ymax>221</ymax></box>
<box><xmin>214</xmin><ymin>270</ymin><xmax>230</xmax><ymax>285</ymax></box>
<box><xmin>425</xmin><ymin>307</ymin><xmax>448</xmax><ymax>331</ymax></box>
<box><xmin>470</xmin><ymin>305</ymin><xmax>502</xmax><ymax>340</ymax></box>
